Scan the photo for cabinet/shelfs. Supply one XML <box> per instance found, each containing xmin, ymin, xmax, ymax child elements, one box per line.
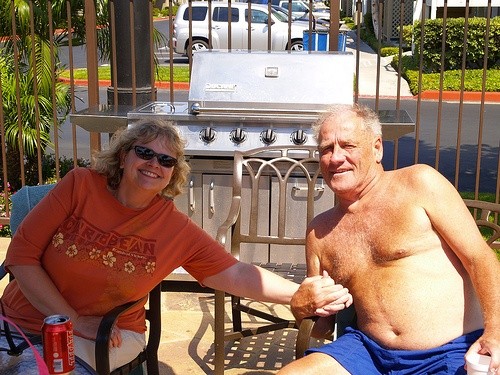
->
<box><xmin>162</xmin><ymin>170</ymin><xmax>270</xmax><ymax>281</ymax></box>
<box><xmin>270</xmin><ymin>174</ymin><xmax>335</xmax><ymax>264</ymax></box>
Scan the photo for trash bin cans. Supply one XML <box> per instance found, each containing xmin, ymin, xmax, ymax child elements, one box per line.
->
<box><xmin>303</xmin><ymin>29</ymin><xmax>348</xmax><ymax>52</ymax></box>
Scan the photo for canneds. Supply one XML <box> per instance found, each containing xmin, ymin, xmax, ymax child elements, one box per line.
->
<box><xmin>41</xmin><ymin>314</ymin><xmax>75</xmax><ymax>375</ymax></box>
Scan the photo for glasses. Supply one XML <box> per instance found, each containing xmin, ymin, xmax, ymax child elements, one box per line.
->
<box><xmin>130</xmin><ymin>145</ymin><xmax>178</xmax><ymax>168</ymax></box>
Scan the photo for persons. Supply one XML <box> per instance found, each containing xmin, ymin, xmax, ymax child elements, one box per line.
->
<box><xmin>0</xmin><ymin>118</ymin><xmax>355</xmax><ymax>375</ymax></box>
<box><xmin>275</xmin><ymin>107</ymin><xmax>500</xmax><ymax>375</ymax></box>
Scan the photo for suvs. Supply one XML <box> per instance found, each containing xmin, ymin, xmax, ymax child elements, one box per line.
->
<box><xmin>167</xmin><ymin>1</ymin><xmax>329</xmax><ymax>61</ymax></box>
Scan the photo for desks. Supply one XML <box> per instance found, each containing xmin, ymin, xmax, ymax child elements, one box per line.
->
<box><xmin>0</xmin><ymin>331</ymin><xmax>98</xmax><ymax>375</ymax></box>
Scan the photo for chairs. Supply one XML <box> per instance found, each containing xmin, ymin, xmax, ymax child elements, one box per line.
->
<box><xmin>215</xmin><ymin>146</ymin><xmax>322</xmax><ymax>375</ymax></box>
<box><xmin>295</xmin><ymin>199</ymin><xmax>500</xmax><ymax>360</ymax></box>
<box><xmin>0</xmin><ymin>184</ymin><xmax>161</xmax><ymax>375</ymax></box>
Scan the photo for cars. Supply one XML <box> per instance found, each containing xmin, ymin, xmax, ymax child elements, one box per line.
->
<box><xmin>259</xmin><ymin>0</ymin><xmax>343</xmax><ymax>32</ymax></box>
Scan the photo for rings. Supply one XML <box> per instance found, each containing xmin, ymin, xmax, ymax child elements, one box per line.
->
<box><xmin>111</xmin><ymin>330</ymin><xmax>114</xmax><ymax>337</ymax></box>
